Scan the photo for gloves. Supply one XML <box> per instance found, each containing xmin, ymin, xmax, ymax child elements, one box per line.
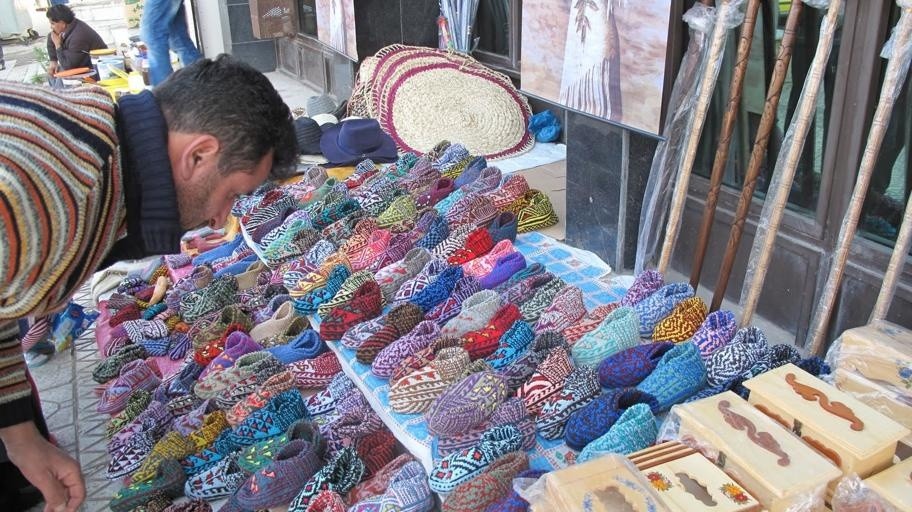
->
<box><xmin>112</xmin><ymin>318</ymin><xmax>168</xmax><ymax>344</ymax></box>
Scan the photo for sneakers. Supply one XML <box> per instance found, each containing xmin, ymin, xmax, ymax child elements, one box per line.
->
<box><xmin>165</xmin><ymin>499</ymin><xmax>214</xmax><ymax>512</ymax></box>
<box><xmin>418</xmin><ymin>176</ymin><xmax>455</xmax><ymax>208</ymax></box>
<box><xmin>731</xmin><ymin>345</ymin><xmax>798</xmax><ymax>391</ymax></box>
<box><xmin>167</xmin><ymin>266</ymin><xmax>212</xmax><ymax>309</ymax></box>
<box><xmin>347</xmin><ymin>463</ymin><xmax>437</xmax><ymax>512</ymax></box>
<box><xmin>305</xmin><ymin>371</ymin><xmax>353</xmax><ymax>414</ymax></box>
<box><xmin>464</xmin><ymin>167</ymin><xmax>501</xmax><ymax>193</ymax></box>
<box><xmin>239</xmin><ymin>442</ymin><xmax>330</xmax><ymax>510</ymax></box>
<box><xmin>151</xmin><ymin>362</ymin><xmax>196</xmax><ymax>401</ymax></box>
<box><xmin>399</xmin><ymin>154</ymin><xmax>433</xmax><ymax>187</ymax></box>
<box><xmin>389</xmin><ymin>208</ymin><xmax>438</xmax><ymax>234</ymax></box>
<box><xmin>563</xmin><ymin>300</ymin><xmax>621</xmax><ymax>347</ymax></box>
<box><xmin>348</xmin><ymin>167</ymin><xmax>390</xmax><ymax>198</ymax></box>
<box><xmin>346</xmin><ymin>169</ymin><xmax>382</xmax><ymax>187</ymax></box>
<box><xmin>621</xmin><ymin>270</ymin><xmax>662</xmax><ymax>306</ymax></box>
<box><xmin>517</xmin><ymin>192</ymin><xmax>558</xmax><ymax>233</ymax></box>
<box><xmin>252</xmin><ymin>207</ymin><xmax>295</xmax><ymax>243</ymax></box>
<box><xmin>290</xmin><ymin>448</ymin><xmax>368</xmax><ymax>511</ymax></box>
<box><xmin>185</xmin><ymin>453</ymin><xmax>246</xmax><ymax>500</ymax></box>
<box><xmin>361</xmin><ymin>176</ymin><xmax>399</xmax><ymax>212</ymax></box>
<box><xmin>502</xmin><ymin>272</ymin><xmax>556</xmax><ymax>304</ymax></box>
<box><xmin>165</xmin><ymin>394</ymin><xmax>196</xmax><ymax>414</ymax></box>
<box><xmin>344</xmin><ymin>453</ymin><xmax>415</xmax><ymax>502</ymax></box>
<box><xmin>353</xmin><ymin>230</ymin><xmax>394</xmax><ymax>273</ymax></box>
<box><xmin>334</xmin><ymin>389</ymin><xmax>366</xmax><ymax>416</ymax></box>
<box><xmin>443</xmin><ymin>452</ymin><xmax>528</xmax><ymax>510</ymax></box>
<box><xmin>435</xmin><ymin>189</ymin><xmax>467</xmax><ymax>214</ymax></box>
<box><xmin>359</xmin><ymin>429</ymin><xmax>399</xmax><ymax>469</ymax></box>
<box><xmin>220</xmin><ymin>256</ymin><xmax>256</xmax><ymax>279</ymax></box>
<box><xmin>454</xmin><ymin>156</ymin><xmax>487</xmax><ymax>188</ymax></box>
<box><xmin>259</xmin><ymin>211</ymin><xmax>311</xmax><ymax>247</ymax></box>
<box><xmin>499</xmin><ymin>189</ymin><xmax>539</xmax><ymax>215</ymax></box>
<box><xmin>182</xmin><ymin>283</ymin><xmax>239</xmax><ymax>324</ymax></box>
<box><xmin>267</xmin><ymin>331</ymin><xmax>323</xmax><ymax>362</ymax></box>
<box><xmin>379</xmin><ymin>195</ymin><xmax>419</xmax><ymax>225</ymax></box>
<box><xmin>250</xmin><ymin>302</ymin><xmax>295</xmax><ymax>341</ymax></box>
<box><xmin>320</xmin><ymin>282</ymin><xmax>381</xmax><ymax>342</ymax></box>
<box><xmin>131</xmin><ymin>431</ymin><xmax>189</xmax><ymax>484</ymax></box>
<box><xmin>106</xmin><ymin>390</ymin><xmax>150</xmax><ymax>436</ymax></box>
<box><xmin>416</xmin><ymin>217</ymin><xmax>454</xmax><ymax>249</ymax></box>
<box><xmin>490</xmin><ymin>212</ymin><xmax>517</xmax><ymax>242</ymax></box>
<box><xmin>284</xmin><ymin>165</ymin><xmax>326</xmax><ymax>194</ymax></box>
<box><xmin>319</xmin><ymin>272</ymin><xmax>374</xmax><ymax>316</ymax></box>
<box><xmin>299</xmin><ymin>176</ymin><xmax>338</xmax><ymax>207</ymax></box>
<box><xmin>459</xmin><ymin>305</ymin><xmax>520</xmax><ymax>360</ymax></box>
<box><xmin>243</xmin><ymin>192</ymin><xmax>284</xmax><ymax>223</ymax></box>
<box><xmin>451</xmin><ymin>198</ymin><xmax>498</xmax><ymax>230</ymax></box>
<box><xmin>230</xmin><ymin>181</ymin><xmax>278</xmax><ymax>216</ymax></box>
<box><xmin>487</xmin><ymin>173</ymin><xmax>512</xmax><ymax>196</ymax></box>
<box><xmin>424</xmin><ymin>278</ymin><xmax>482</xmax><ymax>320</ymax></box>
<box><xmin>435</xmin><ymin>398</ymin><xmax>536</xmax><ymax>452</ymax></box>
<box><xmin>464</xmin><ymin>238</ymin><xmax>514</xmax><ymax>277</ymax></box>
<box><xmin>357</xmin><ymin>302</ymin><xmax>422</xmax><ymax>365</ymax></box>
<box><xmin>225</xmin><ymin>372</ymin><xmax>294</xmax><ymax>426</ymax></box>
<box><xmin>429</xmin><ymin>140</ymin><xmax>450</xmax><ymax>162</ymax></box>
<box><xmin>394</xmin><ymin>259</ymin><xmax>447</xmax><ymax>301</ymax></box>
<box><xmin>324</xmin><ymin>408</ymin><xmax>382</xmax><ymax>445</ymax></box>
<box><xmin>240</xmin><ymin>440</ymin><xmax>318</xmax><ymax>507</ymax></box>
<box><xmin>191</xmin><ymin>411</ymin><xmax>225</xmax><ymax>447</ymax></box>
<box><xmin>393</xmin><ymin>152</ymin><xmax>419</xmax><ymax>176</ymax></box>
<box><xmin>261</xmin><ymin>316</ymin><xmax>314</xmax><ymax>349</ymax></box>
<box><xmin>434</xmin><ymin>144</ymin><xmax>463</xmax><ymax>167</ymax></box>
<box><xmin>481</xmin><ymin>322</ymin><xmax>536</xmax><ymax>369</ymax></box>
<box><xmin>573</xmin><ymin>307</ymin><xmax>639</xmax><ymax>368</ymax></box>
<box><xmin>308</xmin><ymin>182</ymin><xmax>348</xmax><ymax>212</ymax></box>
<box><xmin>322</xmin><ymin>210</ymin><xmax>374</xmax><ymax>245</ymax></box>
<box><xmin>388</xmin><ymin>347</ymin><xmax>472</xmax><ymax>413</ymax></box>
<box><xmin>498</xmin><ymin>263</ymin><xmax>545</xmax><ymax>293</ymax></box>
<box><xmin>428</xmin><ymin>424</ymin><xmax>525</xmax><ymax>494</ymax></box>
<box><xmin>686</xmin><ymin>375</ymin><xmax>750</xmax><ymax>404</ymax></box>
<box><xmin>799</xmin><ymin>360</ymin><xmax>831</xmax><ymax>377</ymax></box>
<box><xmin>287</xmin><ymin>351</ymin><xmax>342</xmax><ymax>382</ymax></box>
<box><xmin>434</xmin><ymin>224</ymin><xmax>478</xmax><ymax>258</ymax></box>
<box><xmin>387</xmin><ymin>338</ymin><xmax>458</xmax><ymax>386</ymax></box>
<box><xmin>247</xmin><ymin>194</ymin><xmax>297</xmax><ymax>234</ymax></box>
<box><xmin>353</xmin><ymin>158</ymin><xmax>375</xmax><ymax>171</ymax></box>
<box><xmin>312</xmin><ymin>198</ymin><xmax>360</xmax><ymax>229</ymax></box>
<box><xmin>440</xmin><ymin>290</ymin><xmax>502</xmax><ymax>338</ymax></box>
<box><xmin>166</xmin><ymin>334</ymin><xmax>192</xmax><ymax>361</ymax></box>
<box><xmin>243</xmin><ymin>273</ymin><xmax>273</xmax><ymax>295</ymax></box>
<box><xmin>286</xmin><ymin>241</ymin><xmax>333</xmax><ymax>285</ymax></box>
<box><xmin>638</xmin><ymin>343</ymin><xmax>709</xmax><ymax>413</ymax></box>
<box><xmin>339</xmin><ymin>218</ymin><xmax>381</xmax><ymax>254</ymax></box>
<box><xmin>440</xmin><ymin>149</ymin><xmax>468</xmax><ymax>174</ymax></box>
<box><xmin>519</xmin><ymin>278</ymin><xmax>564</xmax><ymax>321</ymax></box>
<box><xmin>688</xmin><ymin>311</ymin><xmax>735</xmax><ymax>356</ymax></box>
<box><xmin>93</xmin><ymin>360</ymin><xmax>162</xmax><ymax>413</ymax></box>
<box><xmin>239</xmin><ymin>261</ymin><xmax>266</xmax><ymax>285</ymax></box>
<box><xmin>217</xmin><ymin>365</ymin><xmax>286</xmax><ymax>409</ymax></box>
<box><xmin>516</xmin><ymin>348</ymin><xmax>574</xmax><ymax>413</ymax></box>
<box><xmin>410</xmin><ymin>210</ymin><xmax>441</xmax><ymax>237</ymax></box>
<box><xmin>266</xmin><ymin>229</ymin><xmax>319</xmax><ymax>264</ymax></box>
<box><xmin>177</xmin><ymin>400</ymin><xmax>216</xmax><ymax>430</ymax></box>
<box><xmin>480</xmin><ymin>254</ymin><xmax>525</xmax><ymax>289</ymax></box>
<box><xmin>109</xmin><ymin>459</ymin><xmax>183</xmax><ymax>511</ymax></box>
<box><xmin>638</xmin><ymin>283</ymin><xmax>695</xmax><ymax>337</ymax></box>
<box><xmin>493</xmin><ymin>175</ymin><xmax>529</xmax><ymax>206</ymax></box>
<box><xmin>291</xmin><ymin>254</ymin><xmax>350</xmax><ymax>297</ymax></box>
<box><xmin>294</xmin><ymin>266</ymin><xmax>352</xmax><ymax>313</ymax></box>
<box><xmin>565</xmin><ymin>389</ymin><xmax>659</xmax><ymax>448</ymax></box>
<box><xmin>196</xmin><ymin>350</ymin><xmax>269</xmax><ymax>401</ymax></box>
<box><xmin>368</xmin><ymin>235</ymin><xmax>414</xmax><ymax>271</ymax></box>
<box><xmin>340</xmin><ymin>312</ymin><xmax>391</xmax><ymax>353</ymax></box>
<box><xmin>536</xmin><ymin>366</ymin><xmax>603</xmax><ymax>440</ymax></box>
<box><xmin>91</xmin><ymin>345</ymin><xmax>146</xmax><ymax>382</ymax></box>
<box><xmin>197</xmin><ymin>332</ymin><xmax>261</xmax><ymax>381</ymax></box>
<box><xmin>215</xmin><ymin>390</ymin><xmax>308</xmax><ymax>454</ymax></box>
<box><xmin>239</xmin><ymin>418</ymin><xmax>320</xmax><ymax>471</ymax></box>
<box><xmin>448</xmin><ymin>226</ymin><xmax>494</xmax><ymax>265</ymax></box>
<box><xmin>28</xmin><ymin>341</ymin><xmax>55</xmax><ymax>354</ymax></box>
<box><xmin>21</xmin><ymin>352</ymin><xmax>49</xmax><ymax>367</ymax></box>
<box><xmin>253</xmin><ymin>294</ymin><xmax>292</xmax><ymax>322</ymax></box>
<box><xmin>499</xmin><ymin>332</ymin><xmax>563</xmax><ymax>386</ymax></box>
<box><xmin>654</xmin><ymin>297</ymin><xmax>706</xmax><ymax>340</ymax></box>
<box><xmin>442</xmin><ymin>155</ymin><xmax>473</xmax><ymax>178</ymax></box>
<box><xmin>598</xmin><ymin>341</ymin><xmax>671</xmax><ymax>387</ymax></box>
<box><xmin>402</xmin><ymin>168</ymin><xmax>440</xmax><ymax>190</ymax></box>
<box><xmin>425</xmin><ymin>360</ymin><xmax>495</xmax><ymax>417</ymax></box>
<box><xmin>447</xmin><ymin>194</ymin><xmax>481</xmax><ymax>218</ymax></box>
<box><xmin>246</xmin><ymin>282</ymin><xmax>288</xmax><ymax>307</ymax></box>
<box><xmin>410</xmin><ymin>266</ymin><xmax>462</xmax><ymax>305</ymax></box>
<box><xmin>219</xmin><ymin>498</ymin><xmax>274</xmax><ymax>511</ymax></box>
<box><xmin>398</xmin><ymin>155</ymin><xmax>398</xmax><ymax>161</ymax></box>
<box><xmin>430</xmin><ymin>373</ymin><xmax>508</xmax><ymax>434</ymax></box>
<box><xmin>375</xmin><ymin>248</ymin><xmax>431</xmax><ymax>294</ymax></box>
<box><xmin>534</xmin><ymin>284</ymin><xmax>585</xmax><ymax>334</ymax></box>
<box><xmin>488</xmin><ymin>470</ymin><xmax>552</xmax><ymax>512</ymax></box>
<box><xmin>576</xmin><ymin>404</ymin><xmax>659</xmax><ymax>466</ymax></box>
<box><xmin>709</xmin><ymin>328</ymin><xmax>767</xmax><ymax>385</ymax></box>
<box><xmin>270</xmin><ymin>258</ymin><xmax>300</xmax><ymax>283</ymax></box>
<box><xmin>371</xmin><ymin>320</ymin><xmax>438</xmax><ymax>378</ymax></box>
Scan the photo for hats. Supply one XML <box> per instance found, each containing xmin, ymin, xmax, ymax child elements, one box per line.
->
<box><xmin>319</xmin><ymin>158</ymin><xmax>398</xmax><ymax>168</ymax></box>
<box><xmin>319</xmin><ymin>119</ymin><xmax>398</xmax><ymax>164</ymax></box>
<box><xmin>294</xmin><ymin>117</ymin><xmax>322</xmax><ymax>155</ymax></box>
<box><xmin>305</xmin><ymin>96</ymin><xmax>345</xmax><ymax>115</ymax></box>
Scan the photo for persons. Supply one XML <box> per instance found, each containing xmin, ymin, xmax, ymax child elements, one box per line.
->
<box><xmin>135</xmin><ymin>44</ymin><xmax>148</xmax><ymax>72</ymax></box>
<box><xmin>119</xmin><ymin>42</ymin><xmax>131</xmax><ymax>73</ymax></box>
<box><xmin>139</xmin><ymin>0</ymin><xmax>202</xmax><ymax>87</ymax></box>
<box><xmin>46</xmin><ymin>4</ymin><xmax>108</xmax><ymax>83</ymax></box>
<box><xmin>124</xmin><ymin>43</ymin><xmax>138</xmax><ymax>70</ymax></box>
<box><xmin>0</xmin><ymin>53</ymin><xmax>301</xmax><ymax>512</ymax></box>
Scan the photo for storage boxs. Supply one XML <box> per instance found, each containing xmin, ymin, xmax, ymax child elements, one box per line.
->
<box><xmin>742</xmin><ymin>360</ymin><xmax>912</xmax><ymax>482</ymax></box>
<box><xmin>843</xmin><ymin>319</ymin><xmax>912</xmax><ymax>397</ymax></box>
<box><xmin>831</xmin><ymin>367</ymin><xmax>912</xmax><ymax>429</ymax></box>
<box><xmin>625</xmin><ymin>439</ymin><xmax>761</xmax><ymax>512</ymax></box>
<box><xmin>544</xmin><ymin>454</ymin><xmax>671</xmax><ymax>512</ymax></box>
<box><xmin>862</xmin><ymin>456</ymin><xmax>912</xmax><ymax>512</ymax></box>
<box><xmin>671</xmin><ymin>388</ymin><xmax>843</xmax><ymax>512</ymax></box>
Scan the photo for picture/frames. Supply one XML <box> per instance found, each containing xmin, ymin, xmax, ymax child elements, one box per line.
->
<box><xmin>514</xmin><ymin>1</ymin><xmax>672</xmax><ymax>142</ymax></box>
